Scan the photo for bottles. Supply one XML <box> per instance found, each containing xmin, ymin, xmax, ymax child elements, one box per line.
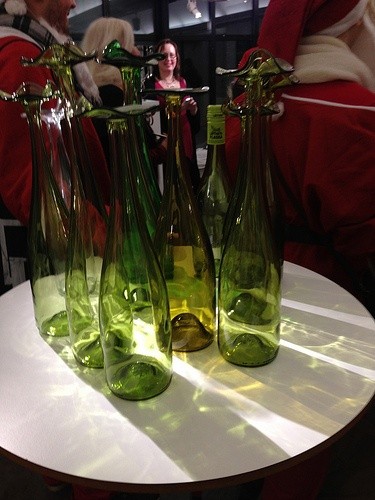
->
<box><xmin>74</xmin><ymin>34</ymin><xmax>172</xmax><ymax>401</ymax></box>
<box><xmin>214</xmin><ymin>46</ymin><xmax>300</xmax><ymax>367</ymax></box>
<box><xmin>0</xmin><ymin>38</ymin><xmax>100</xmax><ymax>338</ymax></box>
<box><xmin>197</xmin><ymin>104</ymin><xmax>232</xmax><ymax>279</ymax></box>
<box><xmin>152</xmin><ymin>85</ymin><xmax>216</xmax><ymax>353</ymax></box>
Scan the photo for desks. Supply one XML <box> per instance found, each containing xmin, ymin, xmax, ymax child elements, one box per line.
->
<box><xmin>0</xmin><ymin>259</ymin><xmax>375</xmax><ymax>500</ymax></box>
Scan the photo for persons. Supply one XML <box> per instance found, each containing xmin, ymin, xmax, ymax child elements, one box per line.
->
<box><xmin>145</xmin><ymin>38</ymin><xmax>202</xmax><ymax>245</ymax></box>
<box><xmin>1</xmin><ymin>0</ymin><xmax>120</xmax><ymax>294</ymax></box>
<box><xmin>73</xmin><ymin>17</ymin><xmax>168</xmax><ymax>200</ymax></box>
<box><xmin>223</xmin><ymin>0</ymin><xmax>375</xmax><ymax>321</ymax></box>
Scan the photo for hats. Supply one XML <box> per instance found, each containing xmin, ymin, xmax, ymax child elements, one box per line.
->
<box><xmin>256</xmin><ymin>0</ymin><xmax>374</xmax><ymax>133</ymax></box>
